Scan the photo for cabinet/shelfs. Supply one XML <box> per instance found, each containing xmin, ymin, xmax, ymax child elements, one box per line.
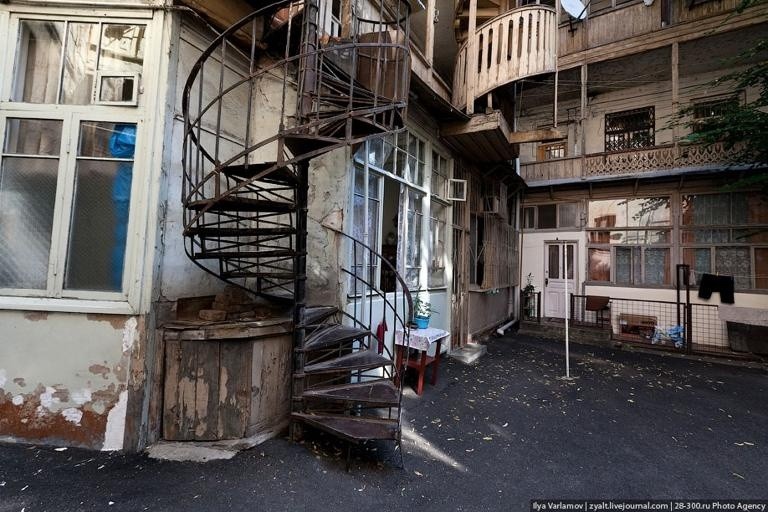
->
<box><xmin>521</xmin><ymin>289</ymin><xmax>541</xmax><ymax>322</ymax></box>
<box><xmin>596</xmin><ymin>302</ymin><xmax>611</xmax><ymax>328</ymax></box>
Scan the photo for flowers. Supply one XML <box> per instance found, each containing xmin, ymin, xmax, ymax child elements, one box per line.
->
<box><xmin>412</xmin><ymin>285</ymin><xmax>440</xmax><ymax>329</ymax></box>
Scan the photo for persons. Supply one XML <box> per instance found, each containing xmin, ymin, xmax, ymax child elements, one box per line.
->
<box><xmin>383</xmin><ymin>233</ymin><xmax>397</xmax><ymax>269</ymax></box>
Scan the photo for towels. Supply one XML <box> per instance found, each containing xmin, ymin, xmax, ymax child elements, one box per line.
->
<box><xmin>377</xmin><ymin>323</ymin><xmax>387</xmax><ymax>355</ymax></box>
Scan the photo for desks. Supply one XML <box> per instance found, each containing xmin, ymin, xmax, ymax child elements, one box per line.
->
<box><xmin>395</xmin><ymin>328</ymin><xmax>449</xmax><ymax>395</ymax></box>
